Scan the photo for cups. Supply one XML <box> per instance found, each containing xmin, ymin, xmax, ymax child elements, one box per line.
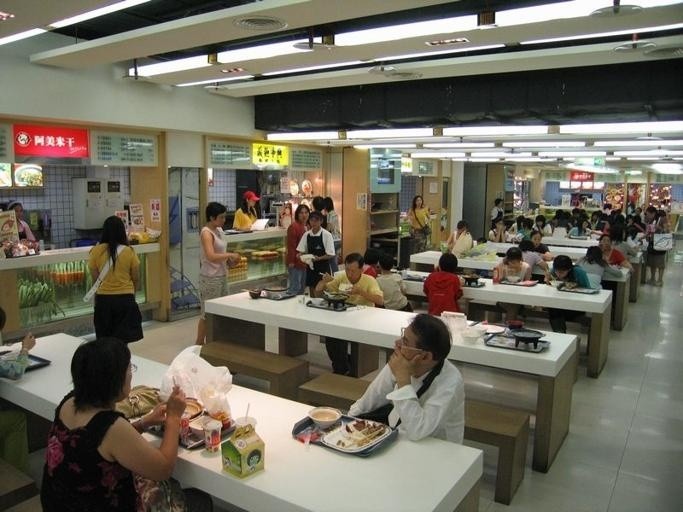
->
<box><xmin>587</xmin><ymin>237</ymin><xmax>590</xmax><ymax>241</ymax></box>
<box><xmin>177</xmin><ymin>414</ymin><xmax>258</xmax><ymax>454</ymax></box>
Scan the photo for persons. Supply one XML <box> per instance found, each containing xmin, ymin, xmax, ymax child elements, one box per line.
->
<box><xmin>88</xmin><ymin>216</ymin><xmax>143</xmax><ymax>343</ymax></box>
<box><xmin>0</xmin><ymin>307</ymin><xmax>35</xmax><ymax>380</ymax></box>
<box><xmin>545</xmin><ymin>199</ymin><xmax>671</xmax><ymax>316</ymax></box>
<box><xmin>401</xmin><ymin>195</ymin><xmax>553</xmax><ymax>316</ymax></box>
<box><xmin>232</xmin><ymin>191</ymin><xmax>261</xmax><ymax>231</ymax></box>
<box><xmin>314</xmin><ymin>253</ymin><xmax>383</xmax><ymax>377</ymax></box>
<box><xmin>285</xmin><ymin>195</ymin><xmax>414</xmax><ymax>314</ymax></box>
<box><xmin>40</xmin><ymin>336</ymin><xmax>212</xmax><ymax>512</ymax></box>
<box><xmin>6</xmin><ymin>199</ymin><xmax>35</xmax><ymax>241</ymax></box>
<box><xmin>195</xmin><ymin>202</ymin><xmax>240</xmax><ymax>345</ymax></box>
<box><xmin>347</xmin><ymin>314</ymin><xmax>464</xmax><ymax>444</ymax></box>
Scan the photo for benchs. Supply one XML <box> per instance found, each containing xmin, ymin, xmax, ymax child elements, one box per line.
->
<box><xmin>298</xmin><ymin>373</ymin><xmax>530</xmax><ymax>506</ymax></box>
<box><xmin>4</xmin><ymin>494</ymin><xmax>43</xmax><ymax>512</ymax></box>
<box><xmin>200</xmin><ymin>341</ymin><xmax>309</xmax><ymax>399</ymax></box>
<box><xmin>0</xmin><ymin>459</ymin><xmax>35</xmax><ymax>512</ymax></box>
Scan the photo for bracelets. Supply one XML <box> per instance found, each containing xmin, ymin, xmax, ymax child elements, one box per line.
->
<box><xmin>20</xmin><ymin>348</ymin><xmax>29</xmax><ymax>353</ymax></box>
<box><xmin>138</xmin><ymin>417</ymin><xmax>149</xmax><ymax>433</ymax></box>
<box><xmin>223</xmin><ymin>253</ymin><xmax>228</xmax><ymax>261</ymax></box>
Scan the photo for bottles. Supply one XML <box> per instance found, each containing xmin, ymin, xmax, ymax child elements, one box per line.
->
<box><xmin>39</xmin><ymin>240</ymin><xmax>44</xmax><ymax>256</ymax></box>
<box><xmin>440</xmin><ymin>311</ymin><xmax>470</xmax><ymax>345</ymax></box>
<box><xmin>592</xmin><ymin>233</ymin><xmax>596</xmax><ymax>242</ymax></box>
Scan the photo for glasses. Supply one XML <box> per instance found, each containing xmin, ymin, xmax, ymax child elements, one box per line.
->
<box><xmin>400</xmin><ymin>327</ymin><xmax>423</xmax><ymax>352</ymax></box>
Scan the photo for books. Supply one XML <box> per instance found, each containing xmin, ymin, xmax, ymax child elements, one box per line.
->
<box><xmin>248</xmin><ymin>218</ymin><xmax>269</xmax><ymax>231</ymax></box>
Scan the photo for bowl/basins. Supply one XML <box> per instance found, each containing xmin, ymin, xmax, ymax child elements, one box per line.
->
<box><xmin>307</xmin><ymin>406</ymin><xmax>343</xmax><ymax>429</ymax></box>
<box><xmin>299</xmin><ymin>253</ymin><xmax>315</xmax><ymax>264</ymax></box>
<box><xmin>549</xmin><ymin>280</ymin><xmax>564</xmax><ymax>287</ymax></box>
<box><xmin>461</xmin><ymin>333</ymin><xmax>480</xmax><ymax>344</ymax></box>
<box><xmin>249</xmin><ymin>289</ymin><xmax>261</xmax><ymax>299</ymax></box>
<box><xmin>505</xmin><ymin>320</ymin><xmax>526</xmax><ymax>331</ymax></box>
<box><xmin>505</xmin><ymin>276</ymin><xmax>521</xmax><ymax>282</ymax></box>
<box><xmin>310</xmin><ymin>298</ymin><xmax>324</xmax><ymax>305</ymax></box>
<box><xmin>161</xmin><ymin>397</ymin><xmax>205</xmax><ymax>430</ymax></box>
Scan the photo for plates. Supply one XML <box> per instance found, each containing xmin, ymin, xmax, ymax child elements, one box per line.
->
<box><xmin>321</xmin><ymin>418</ymin><xmax>393</xmax><ymax>454</ymax></box>
<box><xmin>14</xmin><ymin>164</ymin><xmax>44</xmax><ymax>187</ymax></box>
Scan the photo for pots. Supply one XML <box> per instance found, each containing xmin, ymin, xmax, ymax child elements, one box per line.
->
<box><xmin>320</xmin><ymin>292</ymin><xmax>350</xmax><ymax>302</ymax></box>
<box><xmin>462</xmin><ymin>276</ymin><xmax>480</xmax><ymax>280</ymax></box>
<box><xmin>507</xmin><ymin>330</ymin><xmax>546</xmax><ymax>340</ymax></box>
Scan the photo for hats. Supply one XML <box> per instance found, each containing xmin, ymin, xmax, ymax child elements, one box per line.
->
<box><xmin>242</xmin><ymin>191</ymin><xmax>260</xmax><ymax>201</ymax></box>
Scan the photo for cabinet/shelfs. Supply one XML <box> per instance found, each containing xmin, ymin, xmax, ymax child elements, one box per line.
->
<box><xmin>503</xmin><ymin>191</ymin><xmax>514</xmax><ymax>219</ymax></box>
<box><xmin>368</xmin><ymin>192</ymin><xmax>401</xmax><ymax>270</ymax></box>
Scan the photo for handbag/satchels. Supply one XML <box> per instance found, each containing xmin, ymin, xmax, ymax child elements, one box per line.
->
<box><xmin>422</xmin><ymin>226</ymin><xmax>432</xmax><ymax>236</ymax></box>
<box><xmin>653</xmin><ymin>233</ymin><xmax>673</xmax><ymax>251</ymax></box>
<box><xmin>115</xmin><ymin>384</ymin><xmax>163</xmax><ymax>419</ymax></box>
